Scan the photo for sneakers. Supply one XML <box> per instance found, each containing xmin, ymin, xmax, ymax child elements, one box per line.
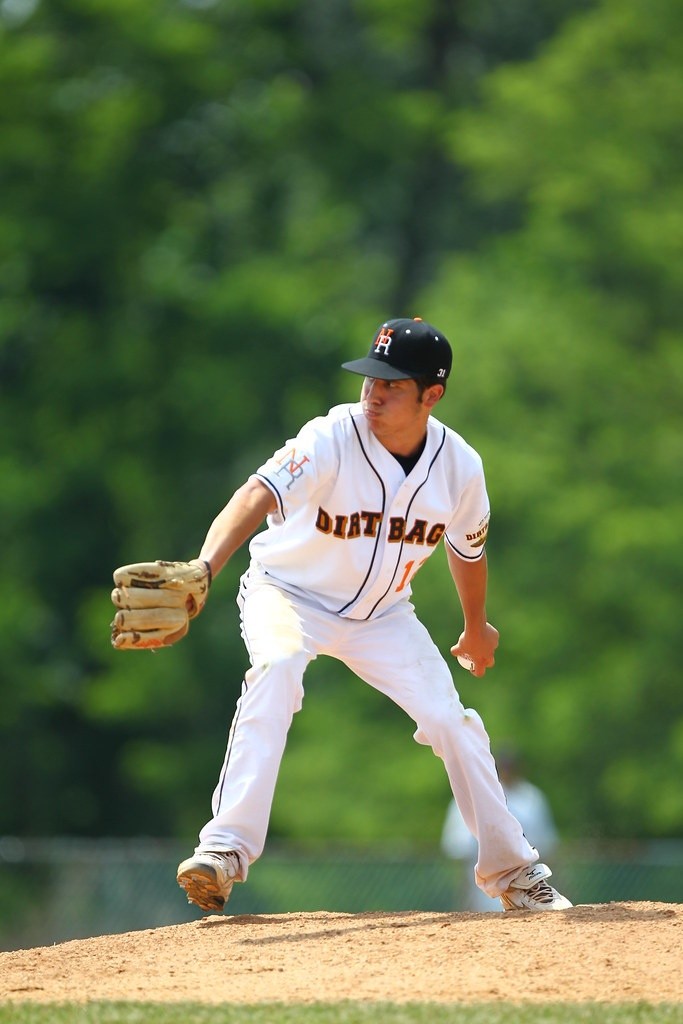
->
<box><xmin>501</xmin><ymin>863</ymin><xmax>573</xmax><ymax>912</ymax></box>
<box><xmin>176</xmin><ymin>851</ymin><xmax>239</xmax><ymax>911</ymax></box>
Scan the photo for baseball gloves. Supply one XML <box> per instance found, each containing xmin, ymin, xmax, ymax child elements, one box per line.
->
<box><xmin>109</xmin><ymin>558</ymin><xmax>213</xmax><ymax>651</ymax></box>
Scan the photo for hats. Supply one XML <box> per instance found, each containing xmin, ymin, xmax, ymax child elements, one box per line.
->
<box><xmin>340</xmin><ymin>317</ymin><xmax>453</xmax><ymax>380</ymax></box>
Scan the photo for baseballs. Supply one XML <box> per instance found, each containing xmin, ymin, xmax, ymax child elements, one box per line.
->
<box><xmin>457</xmin><ymin>652</ymin><xmax>475</xmax><ymax>672</ymax></box>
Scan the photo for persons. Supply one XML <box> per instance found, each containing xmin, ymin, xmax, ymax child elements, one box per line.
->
<box><xmin>108</xmin><ymin>316</ymin><xmax>574</xmax><ymax>912</ymax></box>
